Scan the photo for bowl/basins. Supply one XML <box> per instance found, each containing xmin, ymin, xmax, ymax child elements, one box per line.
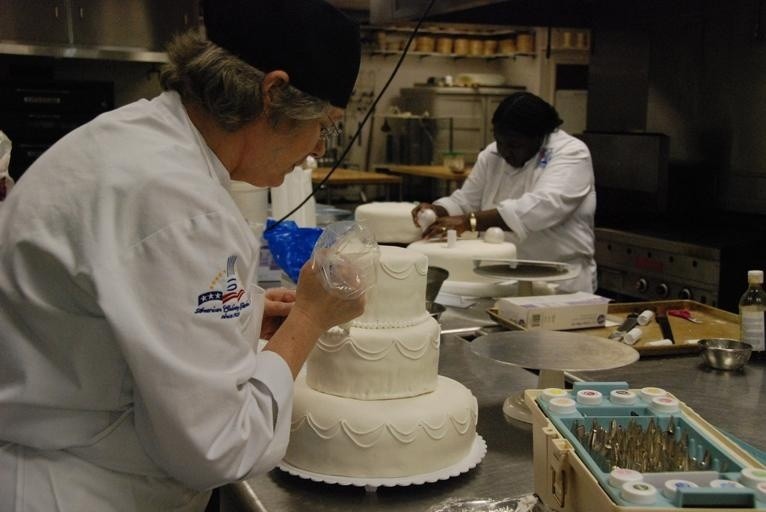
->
<box><xmin>697</xmin><ymin>338</ymin><xmax>753</xmax><ymax>371</ymax></box>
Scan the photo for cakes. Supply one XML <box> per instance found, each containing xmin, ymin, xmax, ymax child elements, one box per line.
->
<box><xmin>356</xmin><ymin>202</ymin><xmax>437</xmax><ymax>243</ymax></box>
<box><xmin>406</xmin><ymin>217</ymin><xmax>517</xmax><ymax>298</ymax></box>
<box><xmin>283</xmin><ymin>245</ymin><xmax>478</xmax><ymax>478</ymax></box>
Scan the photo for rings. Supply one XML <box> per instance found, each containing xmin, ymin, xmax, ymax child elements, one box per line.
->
<box><xmin>442</xmin><ymin>227</ymin><xmax>446</xmax><ymax>233</ymax></box>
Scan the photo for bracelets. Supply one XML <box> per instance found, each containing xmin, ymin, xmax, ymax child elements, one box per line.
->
<box><xmin>468</xmin><ymin>210</ymin><xmax>479</xmax><ymax>232</ymax></box>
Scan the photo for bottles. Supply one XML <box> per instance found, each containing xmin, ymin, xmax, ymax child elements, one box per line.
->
<box><xmin>739</xmin><ymin>270</ymin><xmax>765</xmax><ymax>364</ymax></box>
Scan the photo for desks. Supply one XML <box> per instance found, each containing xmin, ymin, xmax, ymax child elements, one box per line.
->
<box><xmin>310</xmin><ymin>168</ymin><xmax>403</xmax><ymax>205</ymax></box>
<box><xmin>373</xmin><ymin>165</ymin><xmax>472</xmax><ymax>196</ymax></box>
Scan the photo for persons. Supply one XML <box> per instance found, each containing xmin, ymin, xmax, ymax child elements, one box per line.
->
<box><xmin>0</xmin><ymin>0</ymin><xmax>368</xmax><ymax>509</ymax></box>
<box><xmin>411</xmin><ymin>90</ymin><xmax>600</xmax><ymax>296</ymax></box>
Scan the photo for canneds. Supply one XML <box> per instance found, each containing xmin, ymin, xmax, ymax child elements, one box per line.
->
<box><xmin>371</xmin><ymin>31</ymin><xmax>534</xmax><ymax>54</ymax></box>
<box><xmin>560</xmin><ymin>31</ymin><xmax>586</xmax><ymax>48</ymax></box>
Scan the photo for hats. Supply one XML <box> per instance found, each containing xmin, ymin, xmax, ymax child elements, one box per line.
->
<box><xmin>204</xmin><ymin>0</ymin><xmax>361</xmax><ymax>109</ymax></box>
